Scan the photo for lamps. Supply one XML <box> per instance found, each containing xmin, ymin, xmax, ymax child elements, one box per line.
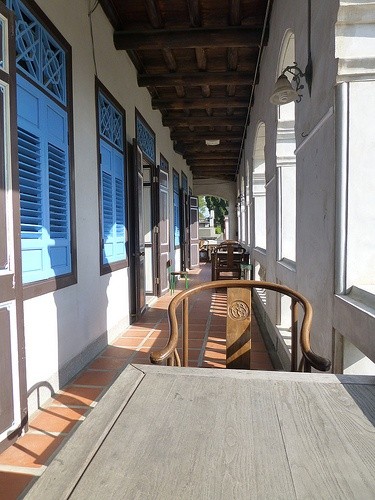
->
<box><xmin>269</xmin><ymin>62</ymin><xmax>305</xmax><ymax>105</ymax></box>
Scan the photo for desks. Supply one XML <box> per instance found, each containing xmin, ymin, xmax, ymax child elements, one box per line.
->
<box><xmin>17</xmin><ymin>363</ymin><xmax>375</xmax><ymax>500</ymax></box>
<box><xmin>211</xmin><ymin>252</ymin><xmax>250</xmax><ymax>281</ymax></box>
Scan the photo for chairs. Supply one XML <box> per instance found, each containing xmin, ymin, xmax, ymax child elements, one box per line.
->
<box><xmin>150</xmin><ymin>240</ymin><xmax>331</xmax><ymax>373</ymax></box>
<box><xmin>213</xmin><ymin>243</ymin><xmax>246</xmax><ymax>281</ymax></box>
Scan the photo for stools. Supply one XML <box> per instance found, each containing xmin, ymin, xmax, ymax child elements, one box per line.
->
<box><xmin>171</xmin><ymin>272</ymin><xmax>188</xmax><ymax>295</ymax></box>
